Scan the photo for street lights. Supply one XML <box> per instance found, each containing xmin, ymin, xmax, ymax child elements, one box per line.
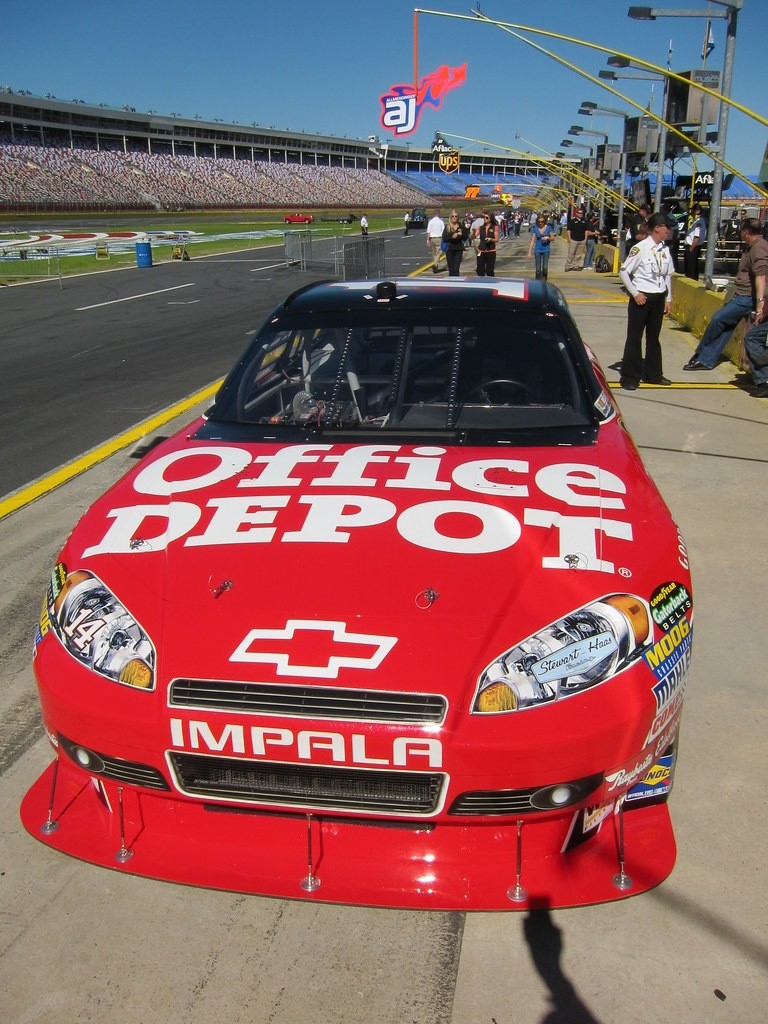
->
<box><xmin>567</xmin><ymin>126</ymin><xmax>609</xmax><ymax>243</ymax></box>
<box><xmin>578</xmin><ymin>101</ymin><xmax>629</xmax><ymax>247</ymax></box>
<box><xmin>628</xmin><ymin>0</ymin><xmax>743</xmax><ymax>286</ymax></box>
<box><xmin>560</xmin><ymin>139</ymin><xmax>595</xmax><ymax>215</ymax></box>
<box><xmin>599</xmin><ymin>55</ymin><xmax>672</xmax><ymax>215</ymax></box>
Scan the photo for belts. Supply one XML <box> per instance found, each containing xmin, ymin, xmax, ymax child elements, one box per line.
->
<box><xmin>474</xmin><ymin>248</ymin><xmax>495</xmax><ymax>256</ymax></box>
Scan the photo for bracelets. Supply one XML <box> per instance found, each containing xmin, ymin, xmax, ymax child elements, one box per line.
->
<box><xmin>426</xmin><ymin>240</ymin><xmax>430</xmax><ymax>242</ymax></box>
<box><xmin>594</xmin><ymin>232</ymin><xmax>595</xmax><ymax>235</ymax></box>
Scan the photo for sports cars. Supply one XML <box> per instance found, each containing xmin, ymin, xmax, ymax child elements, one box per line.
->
<box><xmin>17</xmin><ymin>273</ymin><xmax>695</xmax><ymax>918</ymax></box>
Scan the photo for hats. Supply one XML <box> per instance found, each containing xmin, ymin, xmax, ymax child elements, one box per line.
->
<box><xmin>647</xmin><ymin>213</ymin><xmax>678</xmax><ymax>227</ymax></box>
<box><xmin>687</xmin><ymin>203</ymin><xmax>702</xmax><ymax>211</ymax></box>
<box><xmin>363</xmin><ymin>213</ymin><xmax>368</xmax><ymax>217</ymax></box>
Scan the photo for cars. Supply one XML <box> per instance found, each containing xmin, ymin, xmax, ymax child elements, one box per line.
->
<box><xmin>283</xmin><ymin>213</ymin><xmax>314</xmax><ymax>224</ymax></box>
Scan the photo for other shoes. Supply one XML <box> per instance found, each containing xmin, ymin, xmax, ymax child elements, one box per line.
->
<box><xmin>641</xmin><ymin>374</ymin><xmax>672</xmax><ymax>385</ymax></box>
<box><xmin>504</xmin><ymin>237</ymin><xmax>507</xmax><ymax>239</ymax></box>
<box><xmin>683</xmin><ymin>360</ymin><xmax>710</xmax><ymax>370</ymax></box>
<box><xmin>619</xmin><ymin>375</ymin><xmax>636</xmax><ymax>390</ymax></box>
<box><xmin>583</xmin><ymin>266</ymin><xmax>593</xmax><ymax>270</ymax></box>
<box><xmin>517</xmin><ymin>236</ymin><xmax>520</xmax><ymax>239</ymax></box>
<box><xmin>432</xmin><ymin>265</ymin><xmax>438</xmax><ymax>274</ymax></box>
<box><xmin>749</xmin><ymin>383</ymin><xmax>768</xmax><ymax>397</ymax></box>
<box><xmin>687</xmin><ymin>352</ymin><xmax>699</xmax><ymax>366</ymax></box>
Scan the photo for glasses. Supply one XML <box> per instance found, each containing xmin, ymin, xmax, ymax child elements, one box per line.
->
<box><xmin>482</xmin><ymin>215</ymin><xmax>492</xmax><ymax>219</ymax></box>
<box><xmin>536</xmin><ymin>221</ymin><xmax>546</xmax><ymax>223</ymax></box>
<box><xmin>451</xmin><ymin>215</ymin><xmax>457</xmax><ymax>218</ymax></box>
<box><xmin>578</xmin><ymin>212</ymin><xmax>584</xmax><ymax>214</ymax></box>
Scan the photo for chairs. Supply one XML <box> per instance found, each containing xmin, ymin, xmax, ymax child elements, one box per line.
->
<box><xmin>456</xmin><ymin>332</ymin><xmax>540</xmax><ymax>404</ymax></box>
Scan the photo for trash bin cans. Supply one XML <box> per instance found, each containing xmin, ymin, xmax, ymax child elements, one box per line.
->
<box><xmin>134</xmin><ymin>237</ymin><xmax>154</xmax><ymax>268</ymax></box>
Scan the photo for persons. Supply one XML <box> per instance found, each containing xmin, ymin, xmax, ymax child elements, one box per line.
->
<box><xmin>683</xmin><ymin>217</ymin><xmax>768</xmax><ymax>370</ymax></box>
<box><xmin>618</xmin><ymin>212</ymin><xmax>675</xmax><ymax>391</ymax></box>
<box><xmin>417</xmin><ymin>211</ymin><xmax>422</xmax><ymax>219</ymax></box>
<box><xmin>0</xmin><ymin>144</ymin><xmax>443</xmax><ymax>206</ymax></box>
<box><xmin>402</xmin><ymin>211</ymin><xmax>409</xmax><ymax>236</ymax></box>
<box><xmin>743</xmin><ymin>321</ymin><xmax>768</xmax><ymax>398</ymax></box>
<box><xmin>426</xmin><ymin>203</ymin><xmax>750</xmax><ymax>282</ymax></box>
<box><xmin>361</xmin><ymin>214</ymin><xmax>368</xmax><ymax>240</ymax></box>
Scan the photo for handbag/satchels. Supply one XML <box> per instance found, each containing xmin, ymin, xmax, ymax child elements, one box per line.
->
<box><xmin>595</xmin><ymin>255</ymin><xmax>611</xmax><ymax>273</ymax></box>
<box><xmin>440</xmin><ymin>238</ymin><xmax>450</xmax><ymax>253</ymax></box>
<box><xmin>595</xmin><ymin>234</ymin><xmax>599</xmax><ymax>244</ymax></box>
<box><xmin>625</xmin><ymin>228</ymin><xmax>632</xmax><ymax>241</ymax></box>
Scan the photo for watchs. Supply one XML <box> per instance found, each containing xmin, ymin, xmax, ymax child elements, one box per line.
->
<box><xmin>756</xmin><ymin>297</ymin><xmax>766</xmax><ymax>302</ymax></box>
